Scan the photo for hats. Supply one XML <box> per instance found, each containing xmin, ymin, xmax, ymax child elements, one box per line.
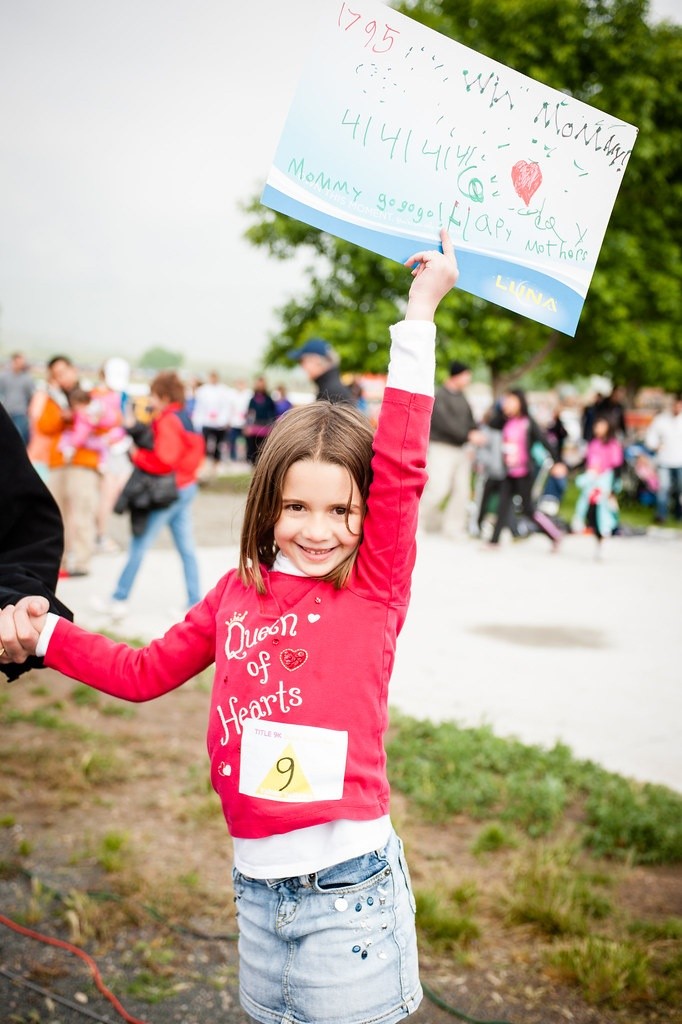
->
<box><xmin>450</xmin><ymin>360</ymin><xmax>470</xmax><ymax>376</ymax></box>
<box><xmin>103</xmin><ymin>358</ymin><xmax>130</xmax><ymax>393</ymax></box>
<box><xmin>288</xmin><ymin>337</ymin><xmax>331</xmax><ymax>360</ymax></box>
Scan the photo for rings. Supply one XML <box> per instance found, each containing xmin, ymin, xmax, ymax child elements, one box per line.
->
<box><xmin>0</xmin><ymin>647</ymin><xmax>4</xmax><ymax>656</ymax></box>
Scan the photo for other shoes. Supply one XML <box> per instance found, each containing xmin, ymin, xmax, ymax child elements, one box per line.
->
<box><xmin>89</xmin><ymin>597</ymin><xmax>129</xmax><ymax>618</ymax></box>
<box><xmin>550</xmin><ymin>534</ymin><xmax>567</xmax><ymax>556</ymax></box>
<box><xmin>480</xmin><ymin>541</ymin><xmax>499</xmax><ymax>551</ymax></box>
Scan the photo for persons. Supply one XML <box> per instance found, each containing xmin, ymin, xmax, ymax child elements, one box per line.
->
<box><xmin>0</xmin><ymin>401</ymin><xmax>74</xmax><ymax>686</ymax></box>
<box><xmin>478</xmin><ymin>387</ymin><xmax>682</xmax><ymax>542</ymax></box>
<box><xmin>421</xmin><ymin>358</ymin><xmax>489</xmax><ymax>542</ymax></box>
<box><xmin>22</xmin><ymin>224</ymin><xmax>459</xmax><ymax>1024</ymax></box>
